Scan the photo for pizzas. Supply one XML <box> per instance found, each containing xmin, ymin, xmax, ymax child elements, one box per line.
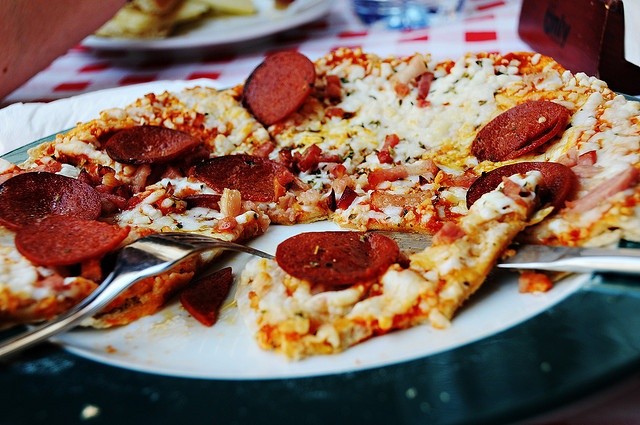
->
<box><xmin>0</xmin><ymin>44</ymin><xmax>635</xmax><ymax>362</ymax></box>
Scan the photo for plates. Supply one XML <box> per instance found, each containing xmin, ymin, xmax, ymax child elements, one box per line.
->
<box><xmin>0</xmin><ymin>94</ymin><xmax>639</xmax><ymax>425</ymax></box>
<box><xmin>78</xmin><ymin>1</ymin><xmax>339</xmax><ymax>49</ymax></box>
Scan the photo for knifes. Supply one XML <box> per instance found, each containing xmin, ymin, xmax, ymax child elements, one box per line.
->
<box><xmin>363</xmin><ymin>227</ymin><xmax>640</xmax><ymax>276</ymax></box>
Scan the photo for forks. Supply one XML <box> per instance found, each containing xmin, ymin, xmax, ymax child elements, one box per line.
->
<box><xmin>1</xmin><ymin>231</ymin><xmax>276</xmax><ymax>359</ymax></box>
